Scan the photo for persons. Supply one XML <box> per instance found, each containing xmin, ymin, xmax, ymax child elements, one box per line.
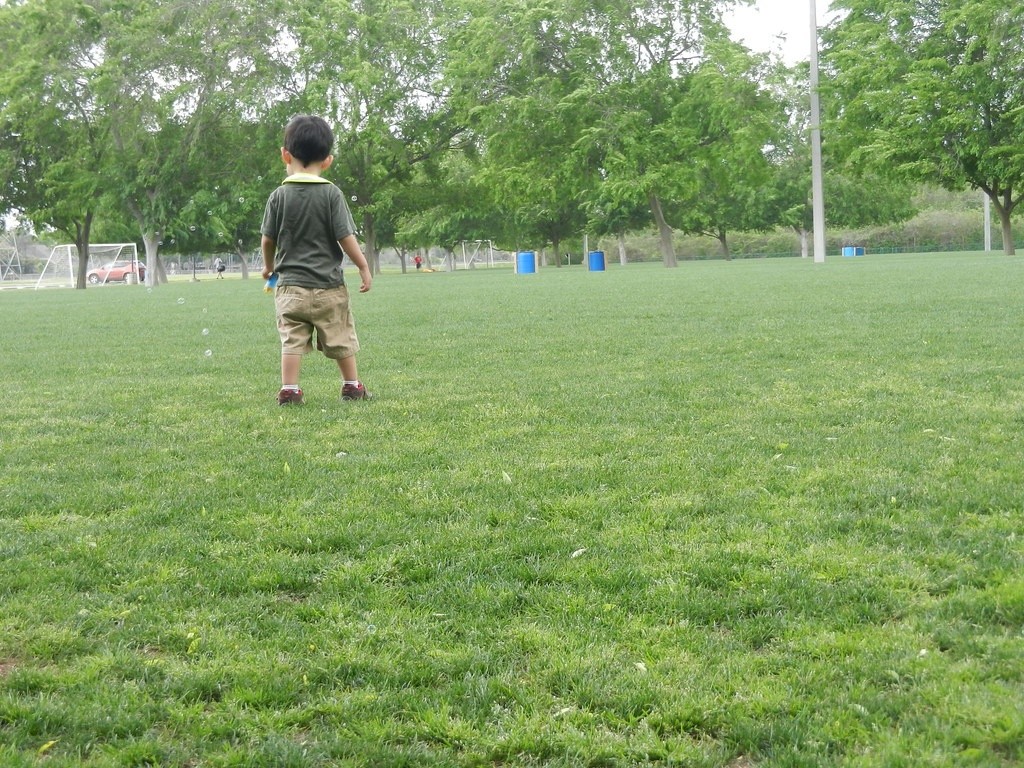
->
<box><xmin>260</xmin><ymin>116</ymin><xmax>372</xmax><ymax>408</ymax></box>
<box><xmin>217</xmin><ymin>261</ymin><xmax>223</xmax><ymax>279</ymax></box>
<box><xmin>415</xmin><ymin>254</ymin><xmax>421</xmax><ymax>270</ymax></box>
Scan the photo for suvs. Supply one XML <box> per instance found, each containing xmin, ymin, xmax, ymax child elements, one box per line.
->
<box><xmin>87</xmin><ymin>260</ymin><xmax>145</xmax><ymax>284</ymax></box>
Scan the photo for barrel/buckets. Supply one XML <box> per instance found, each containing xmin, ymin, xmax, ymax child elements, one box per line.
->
<box><xmin>516</xmin><ymin>251</ymin><xmax>535</xmax><ymax>274</ymax></box>
<box><xmin>589</xmin><ymin>251</ymin><xmax>605</xmax><ymax>271</ymax></box>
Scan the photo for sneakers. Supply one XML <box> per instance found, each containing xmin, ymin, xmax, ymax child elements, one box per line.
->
<box><xmin>276</xmin><ymin>389</ymin><xmax>304</xmax><ymax>409</ymax></box>
<box><xmin>342</xmin><ymin>381</ymin><xmax>373</xmax><ymax>400</ymax></box>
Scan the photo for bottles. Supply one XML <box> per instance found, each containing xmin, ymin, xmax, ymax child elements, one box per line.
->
<box><xmin>263</xmin><ymin>271</ymin><xmax>279</xmax><ymax>293</ymax></box>
